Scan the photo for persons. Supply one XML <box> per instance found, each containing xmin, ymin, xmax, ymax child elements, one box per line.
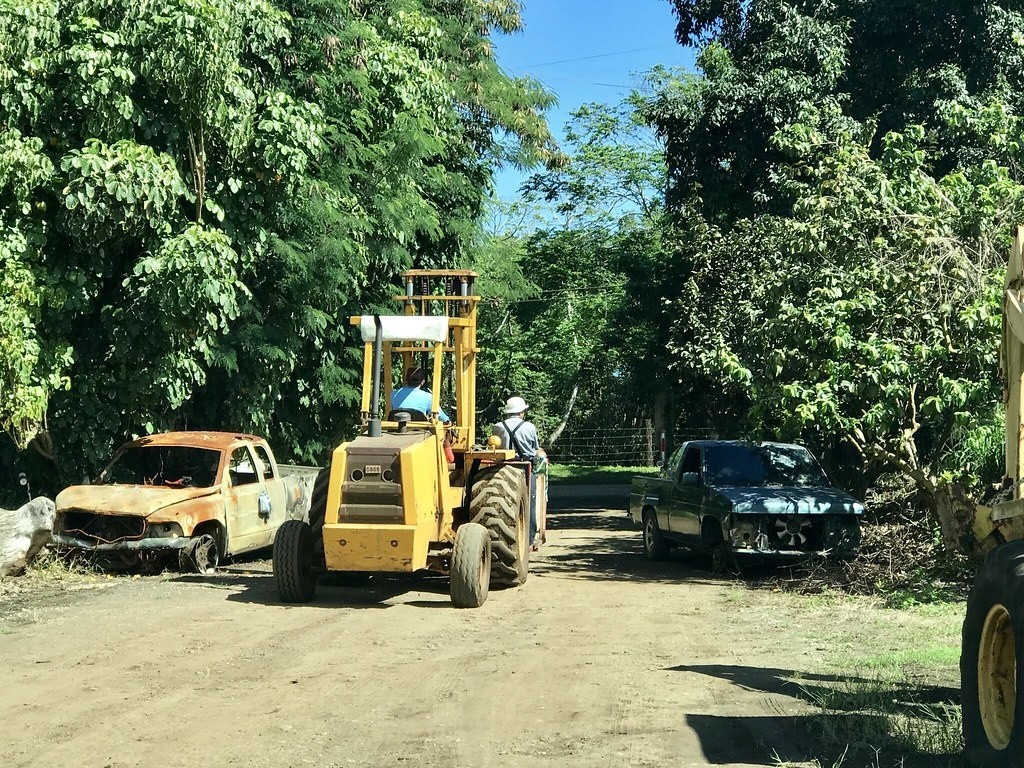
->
<box><xmin>392</xmin><ymin>367</ymin><xmax>451</xmax><ymax>424</ymax></box>
<box><xmin>492</xmin><ymin>397</ymin><xmax>540</xmax><ymax>552</ymax></box>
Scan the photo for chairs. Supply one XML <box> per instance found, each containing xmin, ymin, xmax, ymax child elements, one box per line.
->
<box><xmin>387</xmin><ymin>408</ymin><xmax>429</xmax><ymax>433</ymax></box>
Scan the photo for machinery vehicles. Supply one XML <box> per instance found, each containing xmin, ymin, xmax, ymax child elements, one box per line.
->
<box><xmin>272</xmin><ymin>270</ymin><xmax>546</xmax><ymax>607</ymax></box>
<box><xmin>960</xmin><ymin>223</ymin><xmax>1024</xmax><ymax>768</ymax></box>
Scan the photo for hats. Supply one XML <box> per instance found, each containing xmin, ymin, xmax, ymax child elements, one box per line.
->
<box><xmin>504</xmin><ymin>396</ymin><xmax>529</xmax><ymax>414</ymax></box>
<box><xmin>407</xmin><ymin>367</ymin><xmax>424</xmax><ymax>383</ymax></box>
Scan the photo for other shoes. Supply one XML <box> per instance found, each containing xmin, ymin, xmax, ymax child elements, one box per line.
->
<box><xmin>531</xmin><ymin>539</ymin><xmax>543</xmax><ymax>547</ymax></box>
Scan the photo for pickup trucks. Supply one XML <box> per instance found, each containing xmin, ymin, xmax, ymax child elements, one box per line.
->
<box><xmin>53</xmin><ymin>430</ymin><xmax>323</xmax><ymax>575</ymax></box>
<box><xmin>629</xmin><ymin>439</ymin><xmax>864</xmax><ymax>578</ymax></box>
